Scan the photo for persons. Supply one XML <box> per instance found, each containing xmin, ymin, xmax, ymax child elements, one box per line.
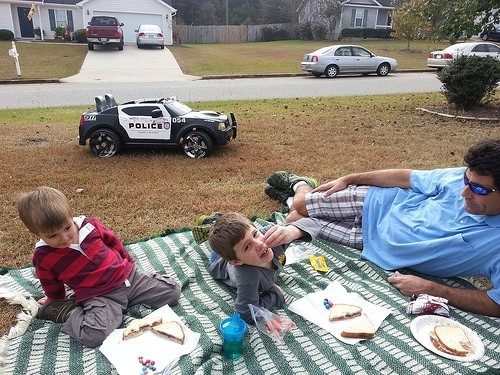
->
<box><xmin>192</xmin><ymin>212</ymin><xmax>321</xmax><ymax>325</ymax></box>
<box><xmin>264</xmin><ymin>139</ymin><xmax>500</xmax><ymax>318</ymax></box>
<box><xmin>18</xmin><ymin>186</ymin><xmax>181</xmax><ymax>347</ymax></box>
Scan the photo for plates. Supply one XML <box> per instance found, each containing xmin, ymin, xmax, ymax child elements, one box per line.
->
<box><xmin>410</xmin><ymin>314</ymin><xmax>485</xmax><ymax>362</ymax></box>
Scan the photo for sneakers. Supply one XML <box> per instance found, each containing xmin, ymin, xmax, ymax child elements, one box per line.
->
<box><xmin>198</xmin><ymin>211</ymin><xmax>223</xmax><ymax>224</ymax></box>
<box><xmin>264</xmin><ymin>185</ymin><xmax>293</xmax><ymax>206</ymax></box>
<box><xmin>192</xmin><ymin>225</ymin><xmax>212</xmax><ymax>245</ymax></box>
<box><xmin>267</xmin><ymin>171</ymin><xmax>317</xmax><ymax>193</ymax></box>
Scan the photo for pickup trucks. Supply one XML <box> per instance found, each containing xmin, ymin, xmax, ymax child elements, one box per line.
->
<box><xmin>86</xmin><ymin>15</ymin><xmax>125</xmax><ymax>51</ymax></box>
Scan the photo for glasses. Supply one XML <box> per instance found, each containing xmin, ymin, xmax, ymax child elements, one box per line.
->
<box><xmin>463</xmin><ymin>168</ymin><xmax>500</xmax><ymax>196</ymax></box>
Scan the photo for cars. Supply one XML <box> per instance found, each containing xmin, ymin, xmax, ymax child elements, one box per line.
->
<box><xmin>479</xmin><ymin>25</ymin><xmax>500</xmax><ymax>42</ymax></box>
<box><xmin>134</xmin><ymin>24</ymin><xmax>165</xmax><ymax>49</ymax></box>
<box><xmin>77</xmin><ymin>93</ymin><xmax>238</xmax><ymax>159</ymax></box>
<box><xmin>300</xmin><ymin>45</ymin><xmax>398</xmax><ymax>79</ymax></box>
<box><xmin>426</xmin><ymin>42</ymin><xmax>500</xmax><ymax>71</ymax></box>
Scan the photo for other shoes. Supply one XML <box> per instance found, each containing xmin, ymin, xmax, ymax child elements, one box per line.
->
<box><xmin>39</xmin><ymin>297</ymin><xmax>79</xmax><ymax>322</ymax></box>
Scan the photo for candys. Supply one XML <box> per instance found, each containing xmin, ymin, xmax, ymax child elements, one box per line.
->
<box><xmin>324</xmin><ymin>298</ymin><xmax>333</xmax><ymax>309</ymax></box>
<box><xmin>138</xmin><ymin>355</ymin><xmax>156</xmax><ymax>375</ymax></box>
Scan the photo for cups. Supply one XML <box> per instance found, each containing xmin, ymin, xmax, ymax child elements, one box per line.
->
<box><xmin>219</xmin><ymin>314</ymin><xmax>248</xmax><ymax>359</ymax></box>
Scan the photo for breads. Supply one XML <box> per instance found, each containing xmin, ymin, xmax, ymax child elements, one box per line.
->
<box><xmin>152</xmin><ymin>321</ymin><xmax>185</xmax><ymax>344</ymax></box>
<box><xmin>329</xmin><ymin>304</ymin><xmax>362</xmax><ymax>322</ymax></box>
<box><xmin>122</xmin><ymin>318</ymin><xmax>163</xmax><ymax>340</ymax></box>
<box><xmin>341</xmin><ymin>315</ymin><xmax>375</xmax><ymax>338</ymax></box>
<box><xmin>428</xmin><ymin>325</ymin><xmax>470</xmax><ymax>356</ymax></box>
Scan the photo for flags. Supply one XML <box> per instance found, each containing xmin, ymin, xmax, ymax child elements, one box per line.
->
<box><xmin>27</xmin><ymin>3</ymin><xmax>37</xmax><ymax>21</ymax></box>
